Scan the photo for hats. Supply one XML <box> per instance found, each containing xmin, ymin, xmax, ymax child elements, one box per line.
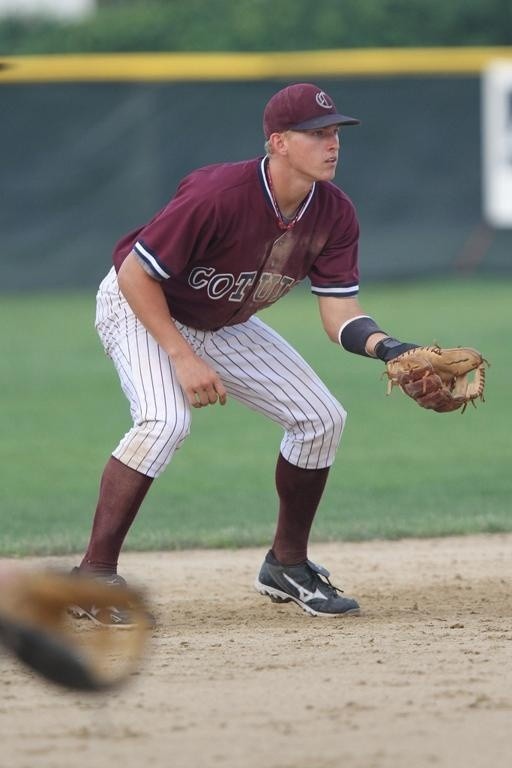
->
<box><xmin>264</xmin><ymin>84</ymin><xmax>359</xmax><ymax>142</ymax></box>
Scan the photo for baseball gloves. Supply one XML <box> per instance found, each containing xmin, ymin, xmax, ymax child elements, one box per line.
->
<box><xmin>6</xmin><ymin>568</ymin><xmax>160</xmax><ymax>692</ymax></box>
<box><xmin>386</xmin><ymin>347</ymin><xmax>485</xmax><ymax>413</ymax></box>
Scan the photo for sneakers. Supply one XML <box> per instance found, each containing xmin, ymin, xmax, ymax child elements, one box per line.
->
<box><xmin>254</xmin><ymin>550</ymin><xmax>360</xmax><ymax>619</ymax></box>
<box><xmin>58</xmin><ymin>566</ymin><xmax>155</xmax><ymax>630</ymax></box>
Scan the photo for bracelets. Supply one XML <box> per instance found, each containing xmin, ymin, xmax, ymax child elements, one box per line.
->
<box><xmin>339</xmin><ymin>315</ymin><xmax>387</xmax><ymax>359</ymax></box>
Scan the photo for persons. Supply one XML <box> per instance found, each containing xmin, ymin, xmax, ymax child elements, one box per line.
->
<box><xmin>62</xmin><ymin>83</ymin><xmax>487</xmax><ymax>633</ymax></box>
